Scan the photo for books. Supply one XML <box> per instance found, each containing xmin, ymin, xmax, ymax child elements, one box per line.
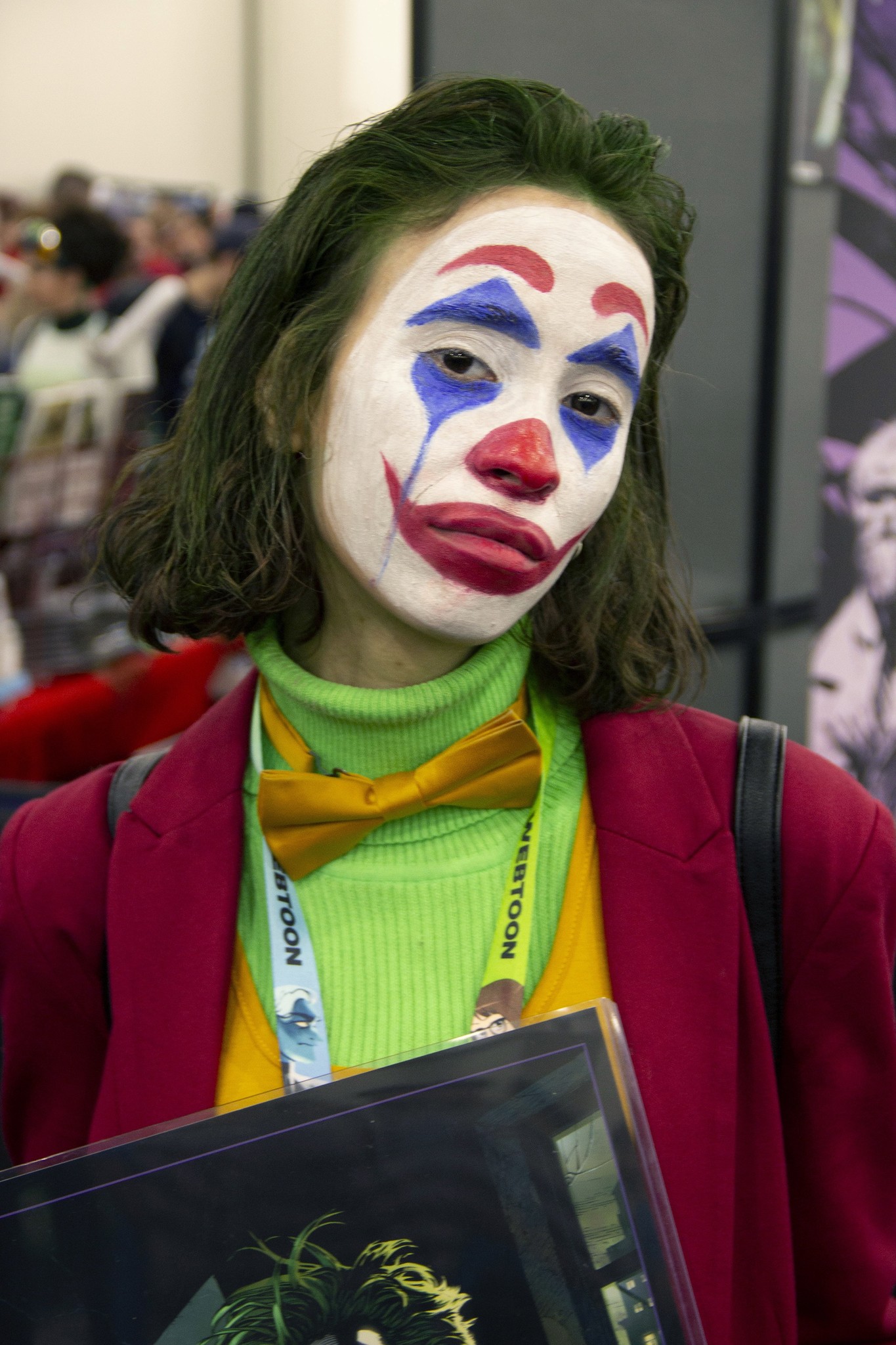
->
<box><xmin>0</xmin><ymin>1002</ymin><xmax>704</xmax><ymax>1345</ymax></box>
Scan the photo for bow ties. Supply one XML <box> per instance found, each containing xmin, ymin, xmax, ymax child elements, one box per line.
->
<box><xmin>254</xmin><ymin>713</ymin><xmax>544</xmax><ymax>883</ymax></box>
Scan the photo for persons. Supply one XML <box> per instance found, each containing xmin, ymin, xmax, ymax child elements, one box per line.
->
<box><xmin>0</xmin><ymin>77</ymin><xmax>895</xmax><ymax>1345</ymax></box>
<box><xmin>0</xmin><ymin>171</ymin><xmax>276</xmax><ymax>795</ymax></box>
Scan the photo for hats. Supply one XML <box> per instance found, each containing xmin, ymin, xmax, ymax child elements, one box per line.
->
<box><xmin>38</xmin><ymin>202</ymin><xmax>126</xmax><ymax>285</ymax></box>
